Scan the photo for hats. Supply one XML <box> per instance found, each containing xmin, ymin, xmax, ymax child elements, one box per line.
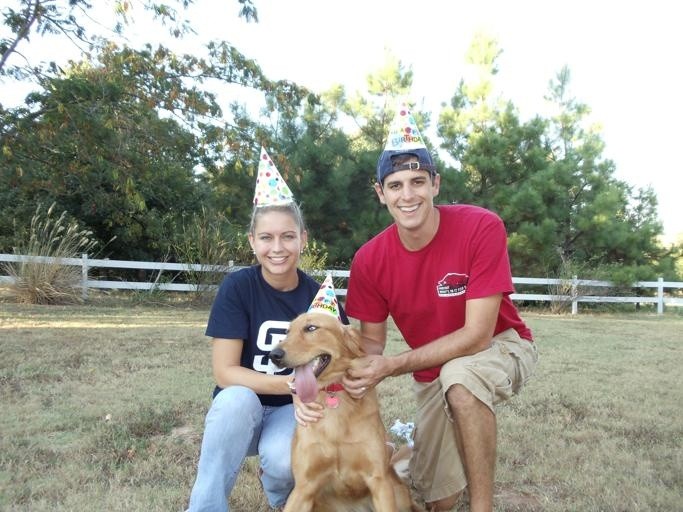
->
<box><xmin>377</xmin><ymin>149</ymin><xmax>436</xmax><ymax>185</ymax></box>
<box><xmin>384</xmin><ymin>95</ymin><xmax>427</xmax><ymax>151</ymax></box>
<box><xmin>253</xmin><ymin>146</ymin><xmax>294</xmax><ymax>208</ymax></box>
<box><xmin>307</xmin><ymin>270</ymin><xmax>342</xmax><ymax>321</ymax></box>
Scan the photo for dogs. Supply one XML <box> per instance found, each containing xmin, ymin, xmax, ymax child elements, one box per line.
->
<box><xmin>267</xmin><ymin>312</ymin><xmax>428</xmax><ymax>512</ymax></box>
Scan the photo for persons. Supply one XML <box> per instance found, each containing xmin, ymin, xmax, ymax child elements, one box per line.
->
<box><xmin>187</xmin><ymin>202</ymin><xmax>352</xmax><ymax>512</ymax></box>
<box><xmin>340</xmin><ymin>150</ymin><xmax>540</xmax><ymax>512</ymax></box>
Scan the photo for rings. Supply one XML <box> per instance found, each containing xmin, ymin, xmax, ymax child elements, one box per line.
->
<box><xmin>362</xmin><ymin>388</ymin><xmax>367</xmax><ymax>392</ymax></box>
